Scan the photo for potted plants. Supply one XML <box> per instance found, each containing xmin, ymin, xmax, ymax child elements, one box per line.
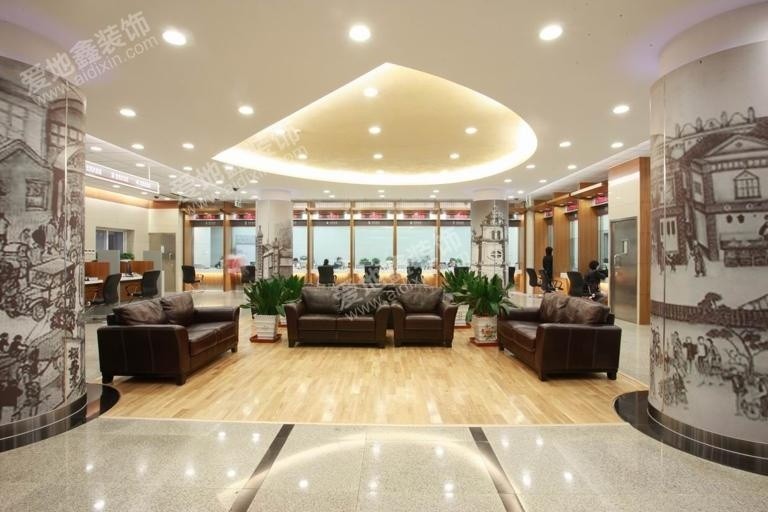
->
<box><xmin>441</xmin><ymin>270</ymin><xmax>471</xmax><ymax>327</ymax></box>
<box><xmin>278</xmin><ymin>274</ymin><xmax>304</xmax><ymax>326</ymax></box>
<box><xmin>241</xmin><ymin>278</ymin><xmax>283</xmax><ymax>340</ymax></box>
<box><xmin>466</xmin><ymin>273</ymin><xmax>504</xmax><ymax>344</ymax></box>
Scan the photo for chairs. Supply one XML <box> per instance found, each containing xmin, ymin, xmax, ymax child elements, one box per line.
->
<box><xmin>390</xmin><ymin>286</ymin><xmax>458</xmax><ymax>347</ymax></box>
<box><xmin>90</xmin><ymin>274</ymin><xmax>122</xmax><ymax>316</ymax></box>
<box><xmin>508</xmin><ymin>266</ymin><xmax>594</xmax><ymax>298</ymax></box>
<box><xmin>318</xmin><ymin>265</ymin><xmax>470</xmax><ymax>285</ymax></box>
<box><xmin>181</xmin><ymin>265</ymin><xmax>200</xmax><ymax>291</ymax></box>
<box><xmin>241</xmin><ymin>266</ymin><xmax>255</xmax><ymax>284</ymax></box>
<box><xmin>125</xmin><ymin>270</ymin><xmax>160</xmax><ymax>303</ymax></box>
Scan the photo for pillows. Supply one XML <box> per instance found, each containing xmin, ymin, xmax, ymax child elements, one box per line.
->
<box><xmin>397</xmin><ymin>286</ymin><xmax>443</xmax><ymax>312</ymax></box>
<box><xmin>341</xmin><ymin>287</ymin><xmax>382</xmax><ymax>315</ymax></box>
<box><xmin>159</xmin><ymin>290</ymin><xmax>194</xmax><ymax>324</ymax></box>
<box><xmin>538</xmin><ymin>292</ymin><xmax>566</xmax><ymax>323</ymax></box>
<box><xmin>113</xmin><ymin>297</ymin><xmax>166</xmax><ymax>325</ymax></box>
<box><xmin>565</xmin><ymin>297</ymin><xmax>610</xmax><ymax>325</ymax></box>
<box><xmin>301</xmin><ymin>286</ymin><xmax>341</xmax><ymax>314</ymax></box>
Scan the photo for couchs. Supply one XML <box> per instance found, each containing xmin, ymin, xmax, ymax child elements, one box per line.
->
<box><xmin>498</xmin><ymin>292</ymin><xmax>621</xmax><ymax>381</ymax></box>
<box><xmin>98</xmin><ymin>294</ymin><xmax>239</xmax><ymax>386</ymax></box>
<box><xmin>284</xmin><ymin>285</ymin><xmax>390</xmax><ymax>348</ymax></box>
